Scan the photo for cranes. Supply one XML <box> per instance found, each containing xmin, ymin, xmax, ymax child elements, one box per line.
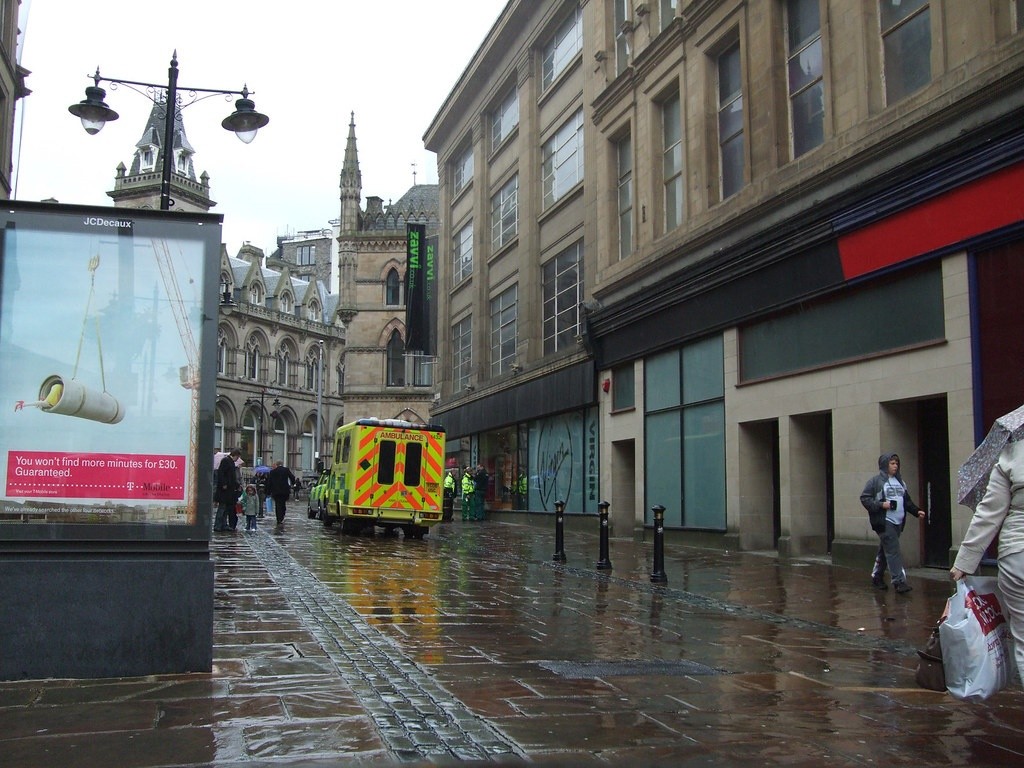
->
<box><xmin>85</xmin><ymin>231</ymin><xmax>201</xmax><ymax>526</ymax></box>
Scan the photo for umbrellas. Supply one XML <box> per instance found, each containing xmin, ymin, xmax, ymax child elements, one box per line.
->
<box><xmin>957</xmin><ymin>403</ymin><xmax>1024</xmax><ymax>519</ymax></box>
<box><xmin>214</xmin><ymin>451</ymin><xmax>244</xmax><ymax>470</ymax></box>
<box><xmin>251</xmin><ymin>465</ymin><xmax>275</xmax><ymax>476</ymax></box>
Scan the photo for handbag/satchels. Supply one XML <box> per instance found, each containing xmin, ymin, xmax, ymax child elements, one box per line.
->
<box><xmin>266</xmin><ymin>496</ymin><xmax>273</xmax><ymax>512</ymax></box>
<box><xmin>938</xmin><ymin>577</ymin><xmax>1015</xmax><ymax>705</ymax></box>
<box><xmin>915</xmin><ymin>578</ymin><xmax>956</xmax><ymax>692</ymax></box>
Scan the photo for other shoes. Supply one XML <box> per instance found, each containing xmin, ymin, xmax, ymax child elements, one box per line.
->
<box><xmin>252</xmin><ymin>528</ymin><xmax>256</xmax><ymax>532</ymax></box>
<box><xmin>873</xmin><ymin>578</ymin><xmax>888</xmax><ymax>589</ymax></box>
<box><xmin>244</xmin><ymin>528</ymin><xmax>250</xmax><ymax>532</ymax></box>
<box><xmin>213</xmin><ymin>524</ymin><xmax>238</xmax><ymax>531</ymax></box>
<box><xmin>893</xmin><ymin>582</ymin><xmax>912</xmax><ymax>592</ymax></box>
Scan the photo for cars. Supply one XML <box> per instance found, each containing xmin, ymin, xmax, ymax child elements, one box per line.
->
<box><xmin>307</xmin><ymin>468</ymin><xmax>331</xmax><ymax>521</ymax></box>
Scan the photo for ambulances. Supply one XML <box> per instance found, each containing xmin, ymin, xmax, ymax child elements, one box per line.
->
<box><xmin>317</xmin><ymin>416</ymin><xmax>447</xmax><ymax>539</ymax></box>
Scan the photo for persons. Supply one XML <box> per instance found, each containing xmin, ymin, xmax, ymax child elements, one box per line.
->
<box><xmin>950</xmin><ymin>438</ymin><xmax>1024</xmax><ymax>689</ymax></box>
<box><xmin>860</xmin><ymin>451</ymin><xmax>925</xmax><ymax>594</ymax></box>
<box><xmin>511</xmin><ymin>470</ymin><xmax>528</xmax><ymax>509</ymax></box>
<box><xmin>443</xmin><ymin>464</ymin><xmax>488</xmax><ymax>522</ymax></box>
<box><xmin>214</xmin><ymin>449</ymin><xmax>302</xmax><ymax>531</ymax></box>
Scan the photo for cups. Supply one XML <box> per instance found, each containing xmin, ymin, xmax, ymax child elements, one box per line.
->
<box><xmin>890</xmin><ymin>500</ymin><xmax>897</xmax><ymax>510</ymax></box>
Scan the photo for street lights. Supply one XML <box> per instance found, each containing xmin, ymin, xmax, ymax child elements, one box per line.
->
<box><xmin>65</xmin><ymin>45</ymin><xmax>272</xmax><ymax>211</ymax></box>
<box><xmin>244</xmin><ymin>390</ymin><xmax>281</xmax><ymax>466</ymax></box>
